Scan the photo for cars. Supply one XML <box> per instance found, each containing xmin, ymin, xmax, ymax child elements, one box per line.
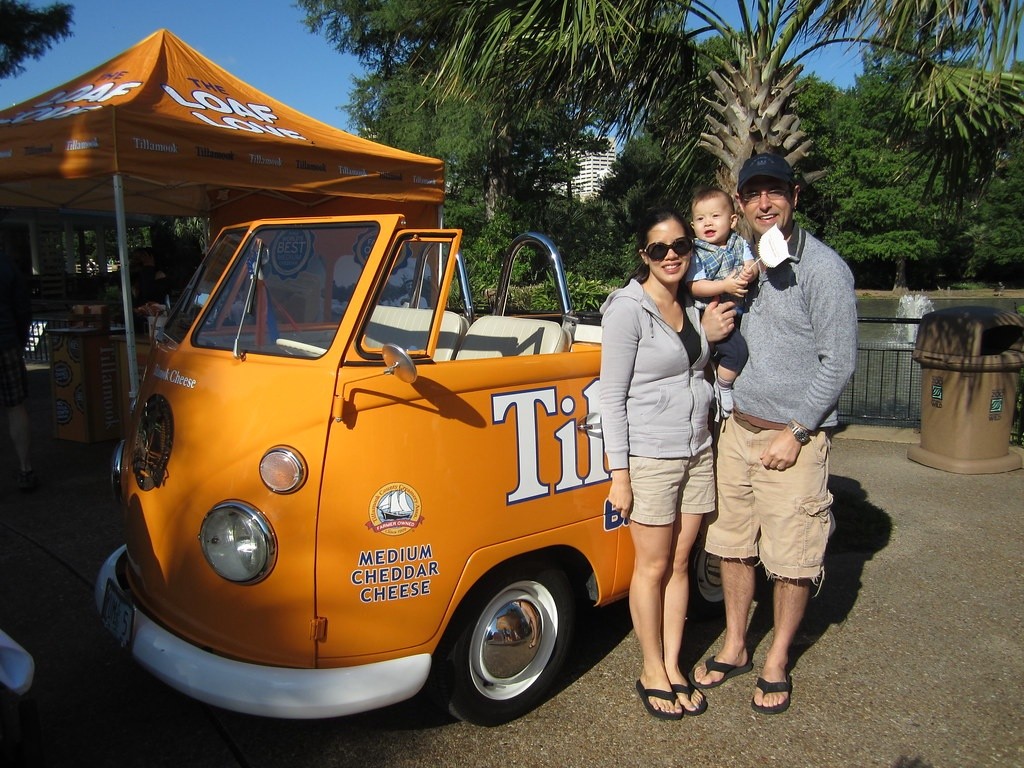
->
<box><xmin>96</xmin><ymin>214</ymin><xmax>725</xmax><ymax>728</ymax></box>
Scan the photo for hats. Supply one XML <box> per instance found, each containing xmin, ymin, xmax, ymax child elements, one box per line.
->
<box><xmin>737</xmin><ymin>153</ymin><xmax>797</xmax><ymax>195</ymax></box>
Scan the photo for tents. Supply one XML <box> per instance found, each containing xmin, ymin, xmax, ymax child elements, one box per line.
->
<box><xmin>1</xmin><ymin>31</ymin><xmax>445</xmax><ymax>419</ymax></box>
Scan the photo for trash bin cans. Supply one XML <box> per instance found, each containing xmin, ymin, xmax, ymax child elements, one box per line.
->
<box><xmin>905</xmin><ymin>304</ymin><xmax>1024</xmax><ymax>475</ymax></box>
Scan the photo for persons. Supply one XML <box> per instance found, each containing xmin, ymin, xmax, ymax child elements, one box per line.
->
<box><xmin>600</xmin><ymin>208</ymin><xmax>708</xmax><ymax>720</ymax></box>
<box><xmin>1</xmin><ymin>241</ymin><xmax>36</xmax><ymax>491</ymax></box>
<box><xmin>688</xmin><ymin>155</ymin><xmax>857</xmax><ymax>714</ymax></box>
<box><xmin>683</xmin><ymin>188</ymin><xmax>759</xmax><ymax>417</ymax></box>
<box><xmin>131</xmin><ymin>222</ymin><xmax>200</xmax><ymax>306</ymax></box>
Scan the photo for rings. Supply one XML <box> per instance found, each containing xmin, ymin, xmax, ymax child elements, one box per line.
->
<box><xmin>776</xmin><ymin>463</ymin><xmax>785</xmax><ymax>470</ymax></box>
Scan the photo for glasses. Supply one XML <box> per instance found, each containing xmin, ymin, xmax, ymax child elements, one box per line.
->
<box><xmin>643</xmin><ymin>237</ymin><xmax>692</xmax><ymax>261</ymax></box>
<box><xmin>739</xmin><ymin>189</ymin><xmax>788</xmax><ymax>202</ymax></box>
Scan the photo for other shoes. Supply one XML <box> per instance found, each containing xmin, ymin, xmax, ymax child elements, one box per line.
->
<box><xmin>11</xmin><ymin>468</ymin><xmax>33</xmax><ymax>490</ymax></box>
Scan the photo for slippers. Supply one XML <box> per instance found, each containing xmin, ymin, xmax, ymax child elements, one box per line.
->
<box><xmin>636</xmin><ymin>678</ymin><xmax>708</xmax><ymax>719</ymax></box>
<box><xmin>689</xmin><ymin>656</ymin><xmax>753</xmax><ymax>689</ymax></box>
<box><xmin>752</xmin><ymin>675</ymin><xmax>792</xmax><ymax>715</ymax></box>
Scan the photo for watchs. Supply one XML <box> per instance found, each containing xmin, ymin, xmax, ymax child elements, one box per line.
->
<box><xmin>787</xmin><ymin>420</ymin><xmax>809</xmax><ymax>443</ymax></box>
